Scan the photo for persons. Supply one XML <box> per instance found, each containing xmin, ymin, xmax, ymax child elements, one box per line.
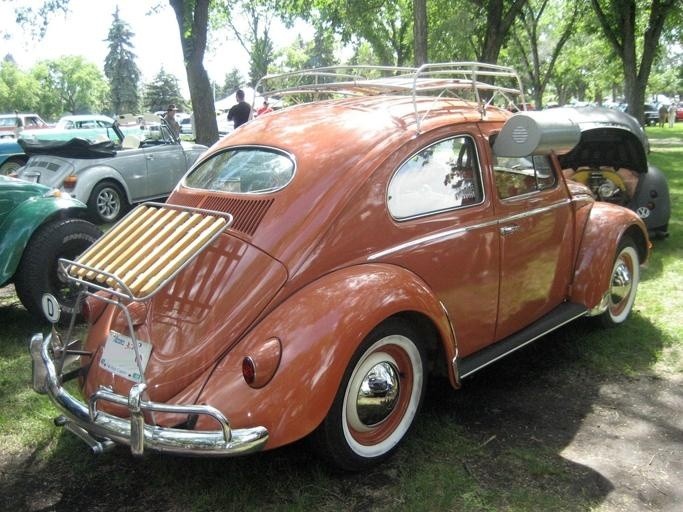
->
<box><xmin>164</xmin><ymin>104</ymin><xmax>180</xmax><ymax>139</ymax></box>
<box><xmin>259</xmin><ymin>100</ymin><xmax>272</xmax><ymax>118</ymax></box>
<box><xmin>658</xmin><ymin>105</ymin><xmax>667</xmax><ymax>128</ymax></box>
<box><xmin>667</xmin><ymin>103</ymin><xmax>677</xmax><ymax>128</ymax></box>
<box><xmin>15</xmin><ymin>110</ymin><xmax>24</xmax><ymax>139</ymax></box>
<box><xmin>227</xmin><ymin>89</ymin><xmax>251</xmax><ymax>130</ymax></box>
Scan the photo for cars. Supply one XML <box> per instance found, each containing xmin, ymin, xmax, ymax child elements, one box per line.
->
<box><xmin>618</xmin><ymin>95</ymin><xmax>671</xmax><ymax>127</ymax></box>
<box><xmin>547</xmin><ymin>105</ymin><xmax>672</xmax><ymax>242</ymax></box>
<box><xmin>141</xmin><ymin>107</ymin><xmax>193</xmax><ymax>136</ymax></box>
<box><xmin>28</xmin><ymin>54</ymin><xmax>654</xmax><ymax>470</ymax></box>
<box><xmin>0</xmin><ymin>111</ymin><xmax>124</xmax><ymax>172</ymax></box>
<box><xmin>0</xmin><ymin>173</ymin><xmax>105</xmax><ymax>327</ymax></box>
<box><xmin>675</xmin><ymin>101</ymin><xmax>683</xmax><ymax>121</ymax></box>
<box><xmin>8</xmin><ymin>116</ymin><xmax>209</xmax><ymax>225</ymax></box>
